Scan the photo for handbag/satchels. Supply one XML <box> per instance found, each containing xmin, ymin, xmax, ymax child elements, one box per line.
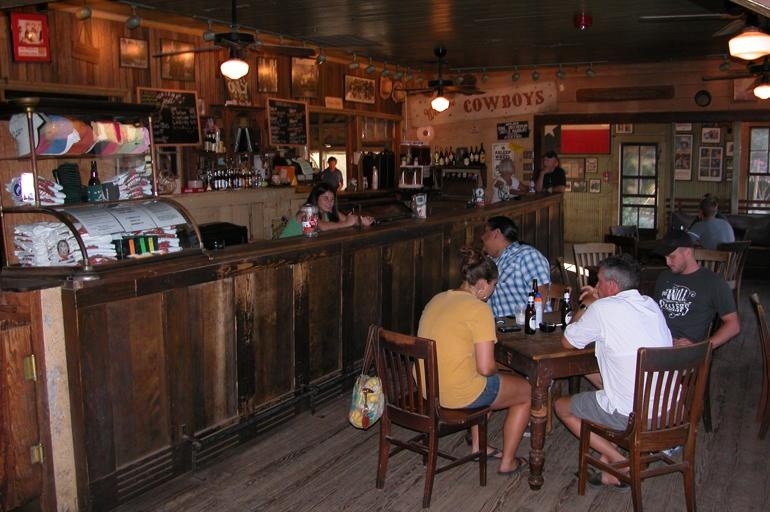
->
<box><xmin>348</xmin><ymin>325</ymin><xmax>386</xmax><ymax>431</ymax></box>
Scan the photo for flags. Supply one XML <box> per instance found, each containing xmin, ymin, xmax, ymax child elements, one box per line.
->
<box><xmin>543</xmin><ymin>124</ymin><xmax>610</xmax><ymax>154</ymax></box>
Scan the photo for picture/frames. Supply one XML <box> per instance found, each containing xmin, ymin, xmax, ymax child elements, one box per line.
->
<box><xmin>159</xmin><ymin>36</ymin><xmax>197</xmax><ymax>84</ymax></box>
<box><xmin>118</xmin><ymin>35</ymin><xmax>151</xmax><ymax>71</ymax></box>
<box><xmin>557</xmin><ymin>122</ymin><xmax>610</xmax><ymax>195</ymax></box>
<box><xmin>256</xmin><ymin>55</ymin><xmax>278</xmax><ymax>95</ymax></box>
<box><xmin>343</xmin><ymin>73</ymin><xmax>376</xmax><ymax>105</ymax></box>
<box><xmin>9</xmin><ymin>11</ymin><xmax>51</xmax><ymax>63</ymax></box>
<box><xmin>614</xmin><ymin>123</ymin><xmax>635</xmax><ymax>135</ymax></box>
<box><xmin>290</xmin><ymin>56</ymin><xmax>321</xmax><ymax>99</ymax></box>
<box><xmin>671</xmin><ymin>122</ymin><xmax>736</xmax><ymax>184</ymax></box>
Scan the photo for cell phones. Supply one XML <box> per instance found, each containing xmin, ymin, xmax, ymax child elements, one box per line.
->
<box><xmin>499</xmin><ymin>325</ymin><xmax>521</xmax><ymax>333</ymax></box>
<box><xmin>507</xmin><ymin>315</ymin><xmax>515</xmax><ymax>319</ymax></box>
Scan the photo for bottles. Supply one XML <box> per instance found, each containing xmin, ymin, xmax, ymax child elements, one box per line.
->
<box><xmin>203</xmin><ymin>131</ymin><xmax>217</xmax><ymax>152</ymax></box>
<box><xmin>372</xmin><ymin>166</ymin><xmax>378</xmax><ymax>190</ymax></box>
<box><xmin>534</xmin><ymin>293</ymin><xmax>543</xmax><ymax>329</ymax></box>
<box><xmin>525</xmin><ymin>292</ymin><xmax>536</xmax><ymax>333</ymax></box>
<box><xmin>531</xmin><ymin>279</ymin><xmax>539</xmax><ymax>293</ymax></box>
<box><xmin>528</xmin><ymin>175</ymin><xmax>535</xmax><ymax>192</ymax></box>
<box><xmin>434</xmin><ymin>141</ymin><xmax>486</xmax><ymax>165</ymax></box>
<box><xmin>561</xmin><ymin>290</ymin><xmax>574</xmax><ymax>329</ymax></box>
<box><xmin>88</xmin><ymin>161</ymin><xmax>103</xmax><ymax>202</ymax></box>
<box><xmin>207</xmin><ymin>168</ymin><xmax>264</xmax><ymax>191</ymax></box>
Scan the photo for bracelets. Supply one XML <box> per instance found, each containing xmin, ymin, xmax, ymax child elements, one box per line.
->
<box><xmin>547</xmin><ymin>187</ymin><xmax>552</xmax><ymax>193</ymax></box>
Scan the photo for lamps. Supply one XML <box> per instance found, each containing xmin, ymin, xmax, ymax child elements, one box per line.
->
<box><xmin>219</xmin><ymin>47</ymin><xmax>250</xmax><ymax>80</ymax></box>
<box><xmin>191</xmin><ymin>14</ymin><xmax>327</xmax><ymax>66</ymax></box>
<box><xmin>728</xmin><ymin>17</ymin><xmax>770</xmax><ymax>62</ymax></box>
<box><xmin>347</xmin><ymin>51</ymin><xmax>429</xmax><ymax>84</ymax></box>
<box><xmin>447</xmin><ymin>58</ymin><xmax>611</xmax><ymax>88</ymax></box>
<box><xmin>1</xmin><ymin>1</ymin><xmax>159</xmax><ymax>30</ymax></box>
<box><xmin>430</xmin><ymin>88</ymin><xmax>449</xmax><ymax>113</ymax></box>
<box><xmin>753</xmin><ymin>76</ymin><xmax>770</xmax><ymax>100</ymax></box>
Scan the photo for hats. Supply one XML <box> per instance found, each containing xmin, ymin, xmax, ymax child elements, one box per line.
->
<box><xmin>655</xmin><ymin>230</ymin><xmax>693</xmax><ymax>256</ymax></box>
<box><xmin>542</xmin><ymin>150</ymin><xmax>556</xmax><ymax>158</ymax></box>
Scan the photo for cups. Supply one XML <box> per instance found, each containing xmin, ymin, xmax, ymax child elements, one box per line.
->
<box><xmin>356</xmin><ymin>176</ymin><xmax>363</xmax><ymax>192</ymax></box>
<box><xmin>514</xmin><ymin>304</ymin><xmax>526</xmax><ymax>325</ymax></box>
<box><xmin>300</xmin><ymin>204</ymin><xmax>320</xmax><ymax>239</ymax></box>
<box><xmin>54</xmin><ymin>163</ymin><xmax>88</xmax><ymax>206</ymax></box>
<box><xmin>101</xmin><ymin>181</ymin><xmax>120</xmax><ymax>201</ymax></box>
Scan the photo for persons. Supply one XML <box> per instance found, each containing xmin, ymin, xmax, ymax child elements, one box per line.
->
<box><xmin>676</xmin><ymin>153</ymin><xmax>689</xmax><ymax>168</ymax></box>
<box><xmin>584</xmin><ymin>231</ymin><xmax>740</xmax><ymax>390</ymax></box>
<box><xmin>279</xmin><ymin>184</ymin><xmax>375</xmax><ymax>239</ymax></box>
<box><xmin>536</xmin><ymin>151</ymin><xmax>565</xmax><ymax>193</ymax></box>
<box><xmin>411</xmin><ymin>247</ymin><xmax>532</xmax><ymax>475</ymax></box>
<box><xmin>687</xmin><ymin>199</ymin><xmax>736</xmax><ymax>280</ymax></box>
<box><xmin>688</xmin><ymin>193</ymin><xmax>728</xmax><ymax>230</ymax></box>
<box><xmin>554</xmin><ymin>254</ymin><xmax>684</xmax><ymax>486</ymax></box>
<box><xmin>676</xmin><ymin>139</ymin><xmax>690</xmax><ymax>153</ymax></box>
<box><xmin>322</xmin><ymin>157</ymin><xmax>343</xmax><ymax>192</ymax></box>
<box><xmin>480</xmin><ymin>216</ymin><xmax>552</xmax><ymax>438</ymax></box>
<box><xmin>491</xmin><ymin>158</ymin><xmax>532</xmax><ymax>205</ymax></box>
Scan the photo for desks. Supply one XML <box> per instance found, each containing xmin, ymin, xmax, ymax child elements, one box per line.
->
<box><xmin>488</xmin><ymin>310</ymin><xmax>597</xmax><ymax>494</ymax></box>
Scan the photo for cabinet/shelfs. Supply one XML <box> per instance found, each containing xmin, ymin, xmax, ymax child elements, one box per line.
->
<box><xmin>1</xmin><ymin>99</ymin><xmax>203</xmax><ymax>275</ymax></box>
<box><xmin>398</xmin><ymin>143</ymin><xmax>432</xmax><ymax>189</ymax></box>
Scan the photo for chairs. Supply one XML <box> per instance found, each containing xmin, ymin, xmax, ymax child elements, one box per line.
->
<box><xmin>730</xmin><ymin>225</ymin><xmax>750</xmax><ymax>241</ymax></box>
<box><xmin>717</xmin><ymin>239</ymin><xmax>750</xmax><ymax>314</ymax></box>
<box><xmin>365</xmin><ymin>321</ymin><xmax>494</xmax><ymax>509</ymax></box>
<box><xmin>580</xmin><ymin>339</ymin><xmax>713</xmax><ymax>512</ymax></box>
<box><xmin>688</xmin><ymin>245</ymin><xmax>731</xmax><ymax>281</ymax></box>
<box><xmin>606</xmin><ymin>224</ymin><xmax>639</xmax><ymax>237</ymax></box>
<box><xmin>750</xmin><ymin>293</ymin><xmax>769</xmax><ymax>439</ymax></box>
<box><xmin>571</xmin><ymin>242</ymin><xmax>617</xmax><ymax>300</ymax></box>
<box><xmin>604</xmin><ymin>233</ymin><xmax>639</xmax><ymax>261</ymax></box>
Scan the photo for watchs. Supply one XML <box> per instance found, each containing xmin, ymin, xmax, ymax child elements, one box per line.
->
<box><xmin>579</xmin><ymin>304</ymin><xmax>587</xmax><ymax>312</ymax></box>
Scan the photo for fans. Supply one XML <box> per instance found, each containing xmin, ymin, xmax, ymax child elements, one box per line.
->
<box><xmin>151</xmin><ymin>1</ymin><xmax>317</xmax><ymax>61</ymax></box>
<box><xmin>701</xmin><ymin>56</ymin><xmax>770</xmax><ymax>90</ymax></box>
<box><xmin>393</xmin><ymin>45</ymin><xmax>487</xmax><ymax>95</ymax></box>
<box><xmin>636</xmin><ymin>1</ymin><xmax>769</xmax><ymax>38</ymax></box>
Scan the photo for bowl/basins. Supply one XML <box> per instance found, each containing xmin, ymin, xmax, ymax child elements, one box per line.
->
<box><xmin>539</xmin><ymin>322</ymin><xmax>557</xmax><ymax>332</ymax></box>
<box><xmin>188</xmin><ymin>180</ymin><xmax>203</xmax><ymax>188</ymax></box>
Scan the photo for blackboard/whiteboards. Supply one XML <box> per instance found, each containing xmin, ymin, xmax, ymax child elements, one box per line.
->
<box><xmin>137</xmin><ymin>87</ymin><xmax>202</xmax><ymax>146</ymax></box>
<box><xmin>266</xmin><ymin>97</ymin><xmax>310</xmax><ymax>147</ymax></box>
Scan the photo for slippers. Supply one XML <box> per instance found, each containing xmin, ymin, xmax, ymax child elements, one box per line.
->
<box><xmin>498</xmin><ymin>456</ymin><xmax>528</xmax><ymax>476</ymax></box>
<box><xmin>473</xmin><ymin>447</ymin><xmax>504</xmax><ymax>462</ymax></box>
<box><xmin>587</xmin><ymin>467</ymin><xmax>632</xmax><ymax>493</ymax></box>
